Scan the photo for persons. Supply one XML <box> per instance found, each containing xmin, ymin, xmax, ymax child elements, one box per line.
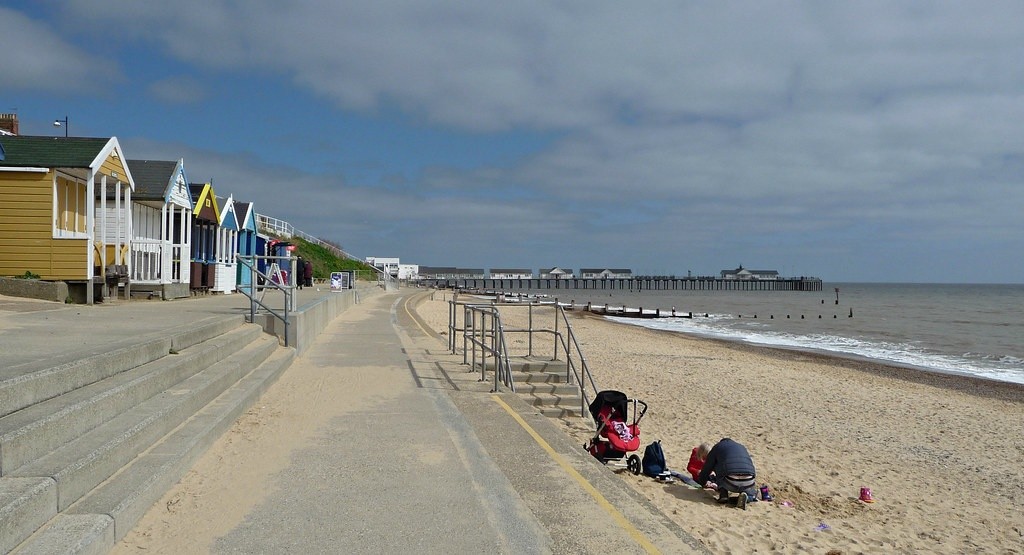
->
<box><xmin>297</xmin><ymin>255</ymin><xmax>313</xmax><ymax>289</ymax></box>
<box><xmin>699</xmin><ymin>437</ymin><xmax>757</xmax><ymax>510</ymax></box>
<box><xmin>686</xmin><ymin>441</ymin><xmax>719</xmax><ymax>490</ymax></box>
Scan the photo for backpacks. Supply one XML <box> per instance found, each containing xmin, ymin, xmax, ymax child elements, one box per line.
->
<box><xmin>642</xmin><ymin>440</ymin><xmax>666</xmax><ymax>479</ymax></box>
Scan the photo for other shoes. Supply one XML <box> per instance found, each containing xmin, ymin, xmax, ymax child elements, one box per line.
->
<box><xmin>296</xmin><ymin>287</ymin><xmax>298</xmax><ymax>289</ymax></box>
<box><xmin>300</xmin><ymin>287</ymin><xmax>302</xmax><ymax>290</ymax></box>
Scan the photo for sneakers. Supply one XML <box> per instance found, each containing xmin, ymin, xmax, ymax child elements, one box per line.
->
<box><xmin>736</xmin><ymin>492</ymin><xmax>748</xmax><ymax>510</ymax></box>
<box><xmin>716</xmin><ymin>492</ymin><xmax>729</xmax><ymax>503</ymax></box>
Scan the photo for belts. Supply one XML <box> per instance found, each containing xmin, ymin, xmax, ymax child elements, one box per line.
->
<box><xmin>724</xmin><ymin>478</ymin><xmax>756</xmax><ymax>490</ymax></box>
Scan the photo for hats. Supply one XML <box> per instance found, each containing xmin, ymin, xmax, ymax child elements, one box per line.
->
<box><xmin>297</xmin><ymin>255</ymin><xmax>301</xmax><ymax>257</ymax></box>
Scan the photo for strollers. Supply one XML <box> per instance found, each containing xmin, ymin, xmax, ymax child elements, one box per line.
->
<box><xmin>583</xmin><ymin>390</ymin><xmax>647</xmax><ymax>476</ymax></box>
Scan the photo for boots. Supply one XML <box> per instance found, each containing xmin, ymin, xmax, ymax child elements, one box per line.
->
<box><xmin>760</xmin><ymin>485</ymin><xmax>776</xmax><ymax>501</ymax></box>
<box><xmin>859</xmin><ymin>488</ymin><xmax>875</xmax><ymax>503</ymax></box>
